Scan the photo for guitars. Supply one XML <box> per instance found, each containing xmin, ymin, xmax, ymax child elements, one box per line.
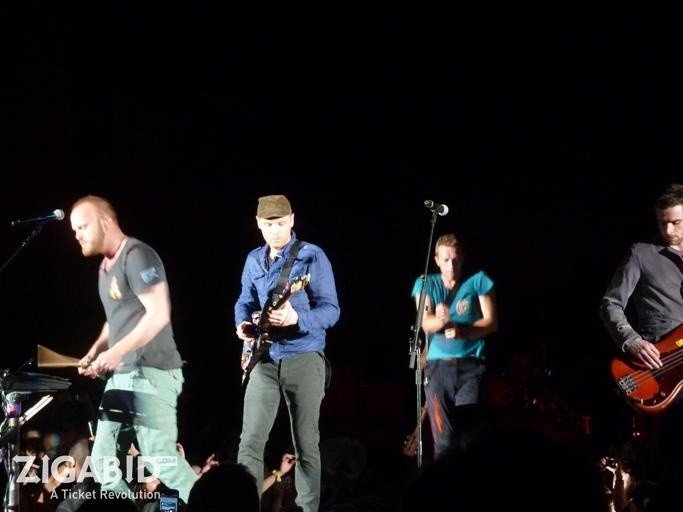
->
<box><xmin>240</xmin><ymin>273</ymin><xmax>310</xmax><ymax>372</ymax></box>
<box><xmin>609</xmin><ymin>324</ymin><xmax>683</xmax><ymax>413</ymax></box>
<box><xmin>403</xmin><ymin>340</ymin><xmax>427</xmax><ymax>456</ymax></box>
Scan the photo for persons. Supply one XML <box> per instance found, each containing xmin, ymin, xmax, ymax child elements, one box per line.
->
<box><xmin>68</xmin><ymin>193</ymin><xmax>200</xmax><ymax>505</ymax></box>
<box><xmin>234</xmin><ymin>190</ymin><xmax>343</xmax><ymax>511</ymax></box>
<box><xmin>406</xmin><ymin>230</ymin><xmax>498</xmax><ymax>461</ymax></box>
<box><xmin>0</xmin><ymin>426</ymin><xmax>454</xmax><ymax>511</ymax></box>
<box><xmin>600</xmin><ymin>177</ymin><xmax>682</xmax><ymax>512</ymax></box>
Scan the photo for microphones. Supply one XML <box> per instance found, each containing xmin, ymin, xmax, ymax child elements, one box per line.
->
<box><xmin>423</xmin><ymin>199</ymin><xmax>449</xmax><ymax>216</ymax></box>
<box><xmin>8</xmin><ymin>208</ymin><xmax>65</xmax><ymax>227</ymax></box>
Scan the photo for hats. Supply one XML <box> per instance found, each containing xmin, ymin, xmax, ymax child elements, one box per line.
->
<box><xmin>255</xmin><ymin>194</ymin><xmax>292</xmax><ymax>220</ymax></box>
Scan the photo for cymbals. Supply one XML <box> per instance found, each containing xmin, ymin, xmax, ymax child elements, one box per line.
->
<box><xmin>0</xmin><ymin>370</ymin><xmax>72</xmax><ymax>392</ymax></box>
